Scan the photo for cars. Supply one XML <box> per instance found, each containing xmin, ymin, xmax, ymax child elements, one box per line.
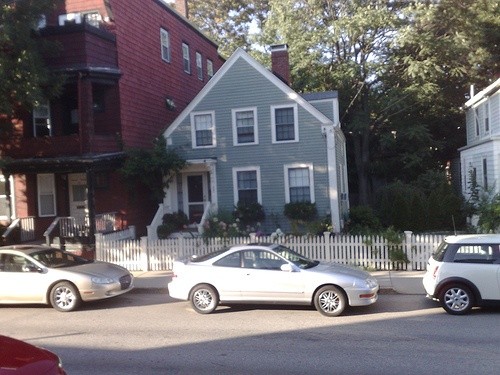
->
<box><xmin>0</xmin><ymin>245</ymin><xmax>134</xmax><ymax>314</ymax></box>
<box><xmin>166</xmin><ymin>242</ymin><xmax>380</xmax><ymax>317</ymax></box>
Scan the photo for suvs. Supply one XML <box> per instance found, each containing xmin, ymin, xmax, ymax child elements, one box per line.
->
<box><xmin>422</xmin><ymin>234</ymin><xmax>500</xmax><ymax>316</ymax></box>
